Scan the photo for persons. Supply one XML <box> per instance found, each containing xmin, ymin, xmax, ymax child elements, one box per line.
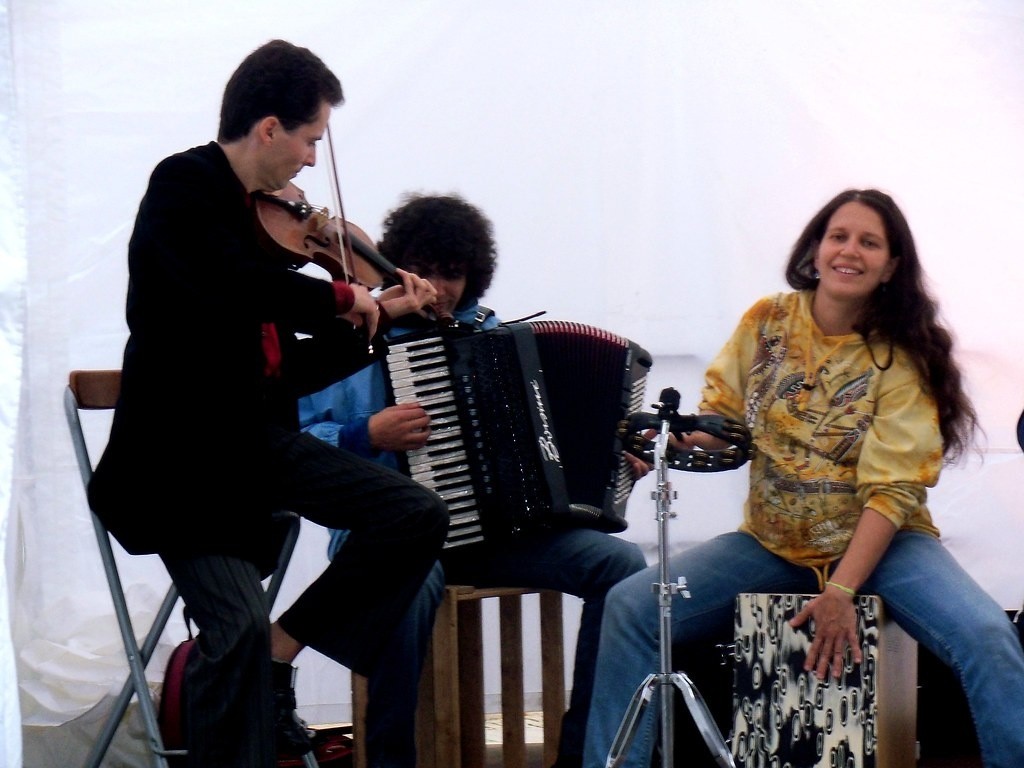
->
<box><xmin>297</xmin><ymin>196</ymin><xmax>658</xmax><ymax>768</ymax></box>
<box><xmin>581</xmin><ymin>189</ymin><xmax>1023</xmax><ymax>768</ymax></box>
<box><xmin>86</xmin><ymin>39</ymin><xmax>450</xmax><ymax>768</ymax></box>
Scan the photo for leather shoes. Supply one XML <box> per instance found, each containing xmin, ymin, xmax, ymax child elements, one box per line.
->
<box><xmin>267</xmin><ymin>659</ymin><xmax>315</xmax><ymax>756</ymax></box>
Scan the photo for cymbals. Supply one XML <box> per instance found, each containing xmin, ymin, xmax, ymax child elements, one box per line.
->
<box><xmin>616</xmin><ymin>410</ymin><xmax>759</xmax><ymax>473</ymax></box>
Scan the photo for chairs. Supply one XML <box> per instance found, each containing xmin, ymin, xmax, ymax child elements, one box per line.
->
<box><xmin>63</xmin><ymin>368</ymin><xmax>303</xmax><ymax>767</ymax></box>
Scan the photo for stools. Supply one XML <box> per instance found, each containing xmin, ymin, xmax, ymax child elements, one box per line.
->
<box><xmin>348</xmin><ymin>581</ymin><xmax>563</xmax><ymax>767</ymax></box>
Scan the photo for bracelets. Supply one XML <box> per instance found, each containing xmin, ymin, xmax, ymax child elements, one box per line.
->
<box><xmin>824</xmin><ymin>580</ymin><xmax>855</xmax><ymax>595</ymax></box>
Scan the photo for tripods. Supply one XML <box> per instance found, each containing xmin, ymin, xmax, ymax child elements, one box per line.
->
<box><xmin>605</xmin><ymin>387</ymin><xmax>737</xmax><ymax>768</ymax></box>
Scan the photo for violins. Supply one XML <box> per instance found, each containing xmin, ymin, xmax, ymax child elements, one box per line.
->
<box><xmin>255</xmin><ymin>179</ymin><xmax>459</xmax><ymax>331</ymax></box>
